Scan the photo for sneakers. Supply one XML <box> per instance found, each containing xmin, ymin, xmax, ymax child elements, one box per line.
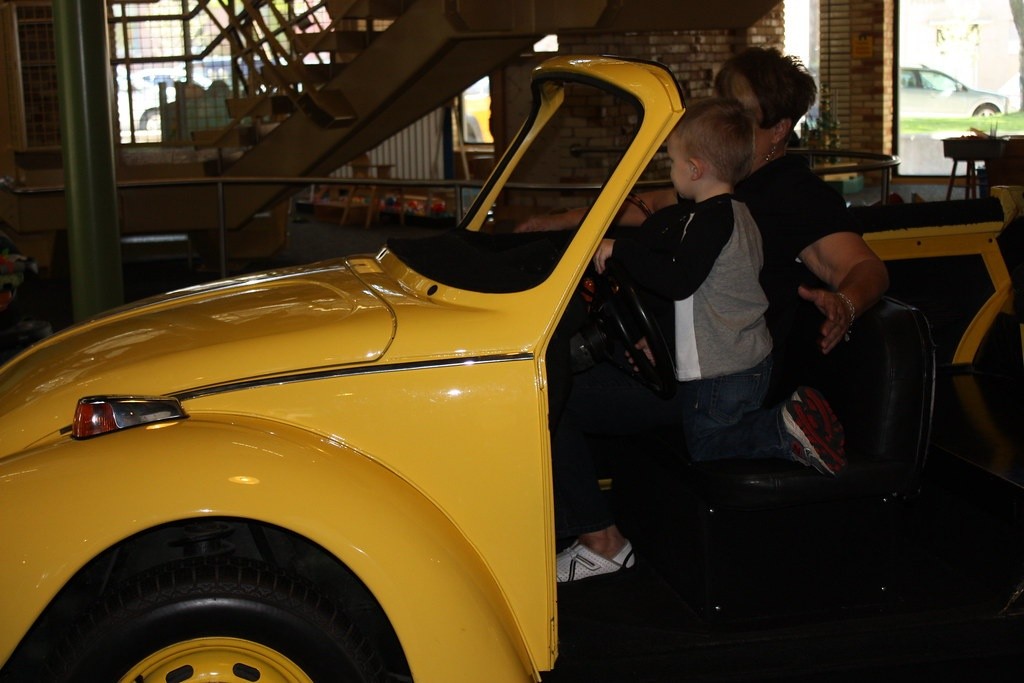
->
<box><xmin>782</xmin><ymin>400</ymin><xmax>846</xmax><ymax>478</ymax></box>
<box><xmin>789</xmin><ymin>385</ymin><xmax>846</xmax><ymax>456</ymax></box>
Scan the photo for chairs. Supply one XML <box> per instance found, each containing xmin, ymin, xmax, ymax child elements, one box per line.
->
<box><xmin>632</xmin><ymin>295</ymin><xmax>937</xmax><ymax>630</ymax></box>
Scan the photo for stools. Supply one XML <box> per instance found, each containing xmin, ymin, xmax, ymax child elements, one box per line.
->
<box><xmin>946</xmin><ymin>160</ymin><xmax>975</xmax><ymax>201</ymax></box>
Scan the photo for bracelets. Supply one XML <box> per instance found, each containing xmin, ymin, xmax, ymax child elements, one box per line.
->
<box><xmin>837</xmin><ymin>292</ymin><xmax>856</xmax><ymax>340</ymax></box>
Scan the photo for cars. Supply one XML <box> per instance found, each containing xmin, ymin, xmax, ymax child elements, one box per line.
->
<box><xmin>895</xmin><ymin>65</ymin><xmax>1006</xmax><ymax>121</ymax></box>
<box><xmin>114</xmin><ymin>66</ymin><xmax>214</xmax><ymax>131</ymax></box>
<box><xmin>178</xmin><ymin>59</ymin><xmax>283</xmax><ymax>94</ymax></box>
<box><xmin>0</xmin><ymin>54</ymin><xmax>1024</xmax><ymax>683</ymax></box>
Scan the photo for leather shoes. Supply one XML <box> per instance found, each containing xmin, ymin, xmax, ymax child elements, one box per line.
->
<box><xmin>554</xmin><ymin>538</ymin><xmax>635</xmax><ymax>584</ymax></box>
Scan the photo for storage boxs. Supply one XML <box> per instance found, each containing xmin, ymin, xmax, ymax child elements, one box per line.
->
<box><xmin>941</xmin><ymin>138</ymin><xmax>1010</xmax><ymax>160</ymax></box>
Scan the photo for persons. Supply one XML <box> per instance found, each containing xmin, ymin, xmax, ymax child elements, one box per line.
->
<box><xmin>595</xmin><ymin>98</ymin><xmax>846</xmax><ymax>477</ymax></box>
<box><xmin>514</xmin><ymin>46</ymin><xmax>890</xmax><ymax>584</ymax></box>
